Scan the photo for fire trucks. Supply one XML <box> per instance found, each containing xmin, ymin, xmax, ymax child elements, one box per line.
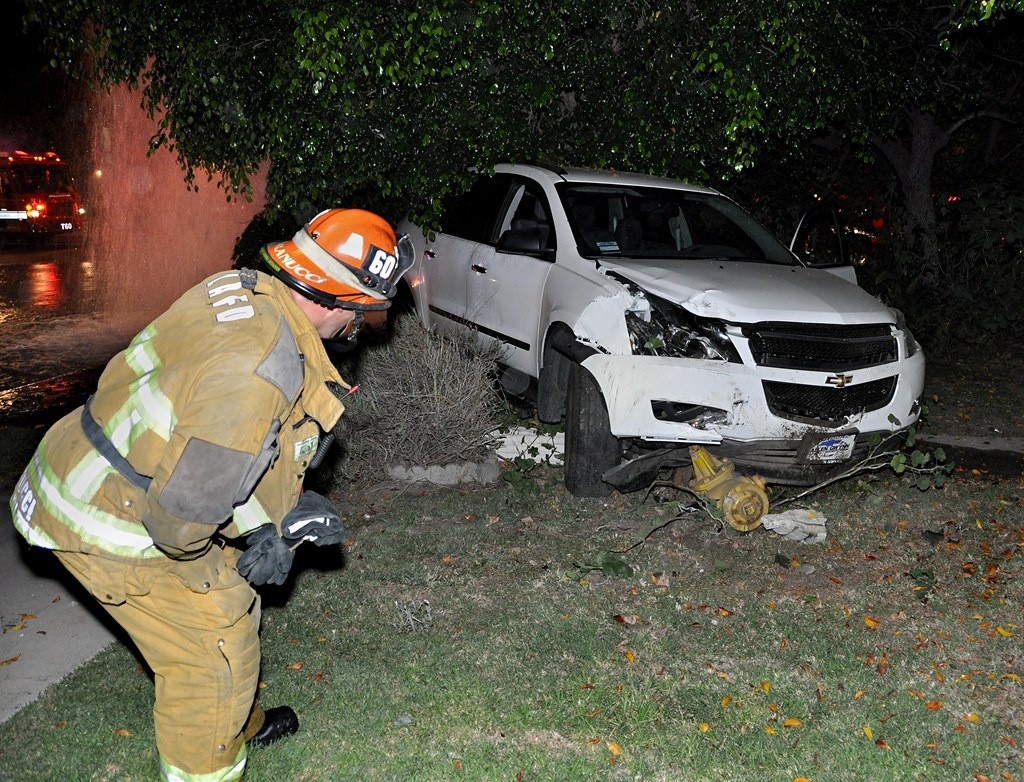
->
<box><xmin>0</xmin><ymin>151</ymin><xmax>85</xmax><ymax>250</ymax></box>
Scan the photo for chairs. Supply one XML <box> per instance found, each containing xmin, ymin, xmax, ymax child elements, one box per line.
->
<box><xmin>613</xmin><ymin>218</ymin><xmax>653</xmax><ymax>256</ymax></box>
<box><xmin>514</xmin><ymin>197</ymin><xmax>550</xmax><ymax>249</ymax></box>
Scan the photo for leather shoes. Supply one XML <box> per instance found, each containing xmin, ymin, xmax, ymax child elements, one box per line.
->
<box><xmin>246</xmin><ymin>705</ymin><xmax>299</xmax><ymax>746</ymax></box>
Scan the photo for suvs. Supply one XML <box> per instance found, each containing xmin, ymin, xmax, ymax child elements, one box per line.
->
<box><xmin>393</xmin><ymin>160</ymin><xmax>926</xmax><ymax>497</ymax></box>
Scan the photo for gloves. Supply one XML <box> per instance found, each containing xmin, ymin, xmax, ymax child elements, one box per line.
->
<box><xmin>284</xmin><ymin>491</ymin><xmax>346</xmax><ymax>546</ymax></box>
<box><xmin>237</xmin><ymin>524</ymin><xmax>295</xmax><ymax>585</ymax></box>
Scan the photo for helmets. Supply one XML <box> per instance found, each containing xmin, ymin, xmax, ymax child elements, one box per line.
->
<box><xmin>261</xmin><ymin>208</ymin><xmax>399</xmax><ymax>311</ymax></box>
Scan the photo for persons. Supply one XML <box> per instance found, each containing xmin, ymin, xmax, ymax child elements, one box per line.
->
<box><xmin>9</xmin><ymin>209</ymin><xmax>415</xmax><ymax>782</ymax></box>
